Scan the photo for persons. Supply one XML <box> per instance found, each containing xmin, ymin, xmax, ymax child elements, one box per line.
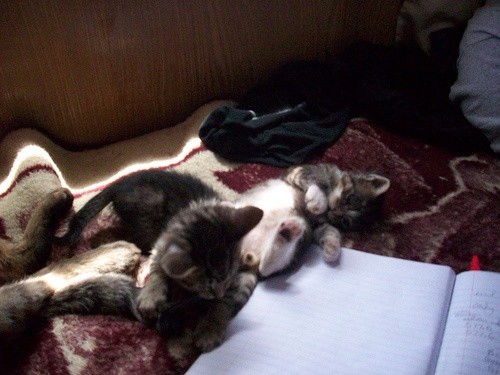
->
<box><xmin>449</xmin><ymin>0</ymin><xmax>500</xmax><ymax>153</ymax></box>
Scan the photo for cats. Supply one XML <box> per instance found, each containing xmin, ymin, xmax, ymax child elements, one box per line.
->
<box><xmin>0</xmin><ymin>188</ymin><xmax>145</xmax><ymax>353</ymax></box>
<box><xmin>54</xmin><ymin>167</ymin><xmax>265</xmax><ymax>354</ymax></box>
<box><xmin>230</xmin><ymin>161</ymin><xmax>391</xmax><ymax>283</ymax></box>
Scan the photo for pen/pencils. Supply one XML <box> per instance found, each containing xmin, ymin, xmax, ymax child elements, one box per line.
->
<box><xmin>469</xmin><ymin>254</ymin><xmax>484</xmax><ymax>271</ymax></box>
